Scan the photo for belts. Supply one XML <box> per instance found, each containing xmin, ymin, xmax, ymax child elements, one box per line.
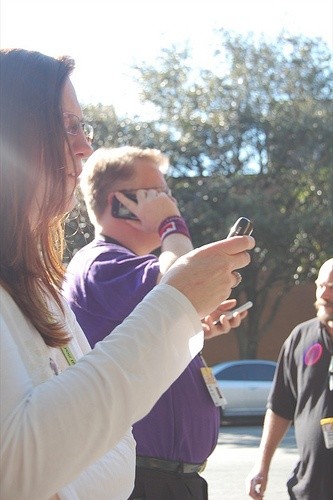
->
<box><xmin>136</xmin><ymin>456</ymin><xmax>206</xmax><ymax>474</ymax></box>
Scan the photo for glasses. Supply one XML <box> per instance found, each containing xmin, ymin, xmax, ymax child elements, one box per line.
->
<box><xmin>62</xmin><ymin>114</ymin><xmax>93</xmax><ymax>140</ymax></box>
<box><xmin>119</xmin><ymin>188</ymin><xmax>170</xmax><ymax>196</ymax></box>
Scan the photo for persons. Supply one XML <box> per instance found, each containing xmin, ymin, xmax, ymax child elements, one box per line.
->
<box><xmin>64</xmin><ymin>147</ymin><xmax>223</xmax><ymax>500</ymax></box>
<box><xmin>0</xmin><ymin>50</ymin><xmax>257</xmax><ymax>496</ymax></box>
<box><xmin>248</xmin><ymin>260</ymin><xmax>333</xmax><ymax>500</ymax></box>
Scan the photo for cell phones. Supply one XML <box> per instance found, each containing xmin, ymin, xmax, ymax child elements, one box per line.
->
<box><xmin>209</xmin><ymin>299</ymin><xmax>256</xmax><ymax>322</ymax></box>
<box><xmin>228</xmin><ymin>215</ymin><xmax>259</xmax><ymax>238</ymax></box>
<box><xmin>110</xmin><ymin>185</ymin><xmax>149</xmax><ymax>223</ymax></box>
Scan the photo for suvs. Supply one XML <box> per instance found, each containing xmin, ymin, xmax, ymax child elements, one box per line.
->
<box><xmin>207</xmin><ymin>359</ymin><xmax>279</xmax><ymax>419</ymax></box>
